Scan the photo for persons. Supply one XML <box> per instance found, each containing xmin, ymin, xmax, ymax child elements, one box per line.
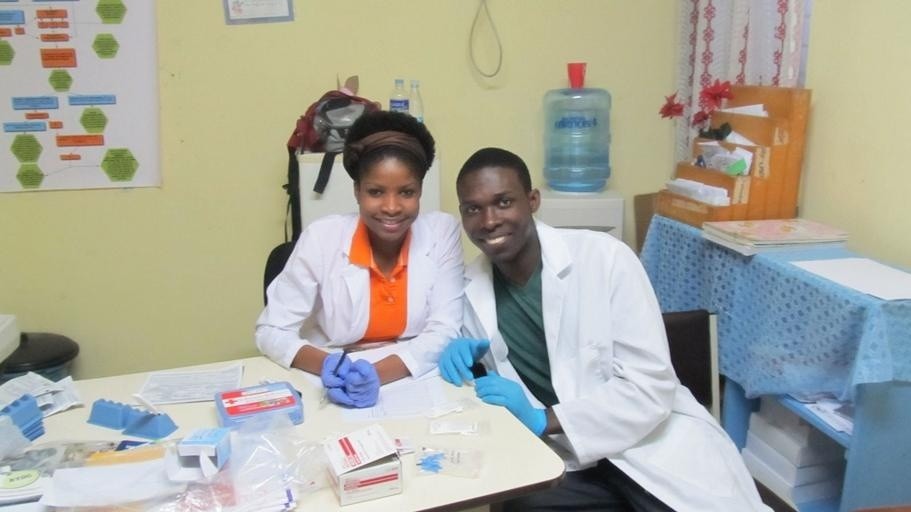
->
<box><xmin>438</xmin><ymin>146</ymin><xmax>772</xmax><ymax>512</ymax></box>
<box><xmin>255</xmin><ymin>113</ymin><xmax>465</xmax><ymax>408</ymax></box>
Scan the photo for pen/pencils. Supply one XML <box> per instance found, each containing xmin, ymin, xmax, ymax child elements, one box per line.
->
<box><xmin>319</xmin><ymin>348</ymin><xmax>351</xmax><ymax>404</ymax></box>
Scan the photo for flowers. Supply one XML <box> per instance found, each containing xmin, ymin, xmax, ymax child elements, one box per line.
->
<box><xmin>658</xmin><ymin>79</ymin><xmax>734</xmax><ymax>140</ymax></box>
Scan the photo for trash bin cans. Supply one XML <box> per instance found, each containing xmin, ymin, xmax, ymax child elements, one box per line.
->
<box><xmin>0</xmin><ymin>331</ymin><xmax>79</xmax><ymax>385</ymax></box>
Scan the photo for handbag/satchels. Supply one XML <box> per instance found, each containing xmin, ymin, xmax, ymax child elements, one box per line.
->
<box><xmin>287</xmin><ymin>89</ymin><xmax>381</xmax><ymax>154</ymax></box>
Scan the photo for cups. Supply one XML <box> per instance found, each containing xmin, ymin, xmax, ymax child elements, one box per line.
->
<box><xmin>567</xmin><ymin>61</ymin><xmax>588</xmax><ymax>89</ymax></box>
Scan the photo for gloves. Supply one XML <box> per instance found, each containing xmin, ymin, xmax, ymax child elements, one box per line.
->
<box><xmin>321</xmin><ymin>352</ymin><xmax>380</xmax><ymax>408</ymax></box>
<box><xmin>474</xmin><ymin>369</ymin><xmax>548</xmax><ymax>437</ymax></box>
<box><xmin>439</xmin><ymin>338</ymin><xmax>490</xmax><ymax>387</ymax></box>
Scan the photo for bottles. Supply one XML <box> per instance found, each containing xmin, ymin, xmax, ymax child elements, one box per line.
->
<box><xmin>389</xmin><ymin>78</ymin><xmax>410</xmax><ymax>114</ymax></box>
<box><xmin>408</xmin><ymin>80</ymin><xmax>426</xmax><ymax>125</ymax></box>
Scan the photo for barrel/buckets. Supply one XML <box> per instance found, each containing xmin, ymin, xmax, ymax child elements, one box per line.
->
<box><xmin>540</xmin><ymin>87</ymin><xmax>613</xmax><ymax>191</ymax></box>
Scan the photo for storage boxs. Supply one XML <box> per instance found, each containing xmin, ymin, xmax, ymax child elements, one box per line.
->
<box><xmin>737</xmin><ymin>393</ymin><xmax>845</xmax><ymax>505</ymax></box>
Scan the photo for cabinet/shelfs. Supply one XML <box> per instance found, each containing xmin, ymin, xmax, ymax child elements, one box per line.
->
<box><xmin>654</xmin><ymin>78</ymin><xmax>812</xmax><ymax>228</ymax></box>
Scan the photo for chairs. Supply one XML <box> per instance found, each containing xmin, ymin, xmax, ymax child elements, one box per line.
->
<box><xmin>661</xmin><ymin>309</ymin><xmax>724</xmax><ymax>429</ymax></box>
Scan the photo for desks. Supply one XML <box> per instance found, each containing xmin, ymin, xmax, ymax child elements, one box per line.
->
<box><xmin>0</xmin><ymin>347</ymin><xmax>568</xmax><ymax>512</ymax></box>
<box><xmin>634</xmin><ymin>214</ymin><xmax>909</xmax><ymax>511</ymax></box>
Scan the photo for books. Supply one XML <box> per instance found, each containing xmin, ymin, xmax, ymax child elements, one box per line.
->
<box><xmin>698</xmin><ymin>218</ymin><xmax>849</xmax><ymax>256</ymax></box>
